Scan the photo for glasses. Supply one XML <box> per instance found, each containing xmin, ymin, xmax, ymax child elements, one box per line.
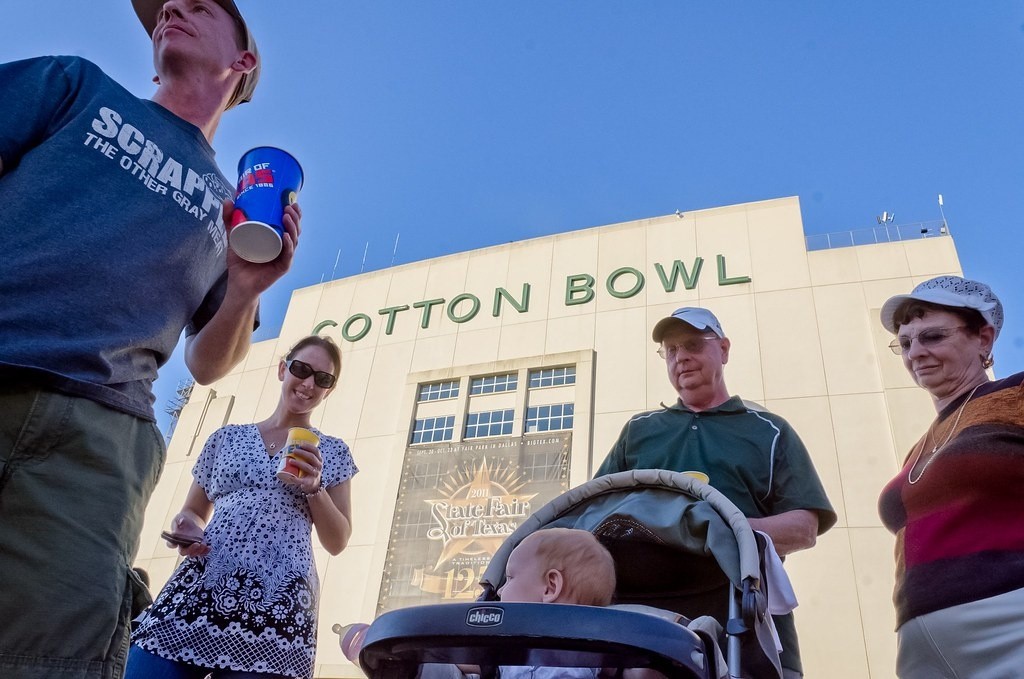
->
<box><xmin>286</xmin><ymin>359</ymin><xmax>335</xmax><ymax>388</ymax></box>
<box><xmin>889</xmin><ymin>325</ymin><xmax>968</xmax><ymax>355</ymax></box>
<box><xmin>657</xmin><ymin>337</ymin><xmax>715</xmax><ymax>359</ymax></box>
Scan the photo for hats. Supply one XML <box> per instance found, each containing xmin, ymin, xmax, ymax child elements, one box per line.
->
<box><xmin>652</xmin><ymin>306</ymin><xmax>723</xmax><ymax>344</ymax></box>
<box><xmin>131</xmin><ymin>0</ymin><xmax>261</xmax><ymax>112</ymax></box>
<box><xmin>879</xmin><ymin>275</ymin><xmax>1004</xmax><ymax>343</ymax></box>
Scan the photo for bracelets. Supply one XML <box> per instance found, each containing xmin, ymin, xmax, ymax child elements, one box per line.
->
<box><xmin>302</xmin><ymin>481</ymin><xmax>324</xmax><ymax>498</ymax></box>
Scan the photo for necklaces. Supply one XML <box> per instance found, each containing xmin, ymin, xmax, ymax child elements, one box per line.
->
<box><xmin>908</xmin><ymin>380</ymin><xmax>989</xmax><ymax>484</ymax></box>
<box><xmin>931</xmin><ymin>394</ymin><xmax>970</xmax><ymax>453</ymax></box>
<box><xmin>270</xmin><ymin>443</ymin><xmax>275</xmax><ymax>449</ymax></box>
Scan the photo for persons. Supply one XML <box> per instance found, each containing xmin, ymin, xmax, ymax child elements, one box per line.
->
<box><xmin>877</xmin><ymin>276</ymin><xmax>1024</xmax><ymax>679</ymax></box>
<box><xmin>0</xmin><ymin>0</ymin><xmax>303</xmax><ymax>679</ymax></box>
<box><xmin>124</xmin><ymin>336</ymin><xmax>359</xmax><ymax>679</ymax></box>
<box><xmin>594</xmin><ymin>306</ymin><xmax>838</xmax><ymax>679</ymax></box>
<box><xmin>454</xmin><ymin>528</ymin><xmax>670</xmax><ymax>679</ymax></box>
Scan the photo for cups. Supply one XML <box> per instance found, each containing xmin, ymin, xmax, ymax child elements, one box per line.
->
<box><xmin>228</xmin><ymin>146</ymin><xmax>305</xmax><ymax>264</ymax></box>
<box><xmin>681</xmin><ymin>471</ymin><xmax>710</xmax><ymax>485</ymax></box>
<box><xmin>276</xmin><ymin>427</ymin><xmax>321</xmax><ymax>484</ymax></box>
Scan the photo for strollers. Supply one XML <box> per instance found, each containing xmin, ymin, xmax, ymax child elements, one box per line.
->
<box><xmin>358</xmin><ymin>469</ymin><xmax>783</xmax><ymax>679</ymax></box>
